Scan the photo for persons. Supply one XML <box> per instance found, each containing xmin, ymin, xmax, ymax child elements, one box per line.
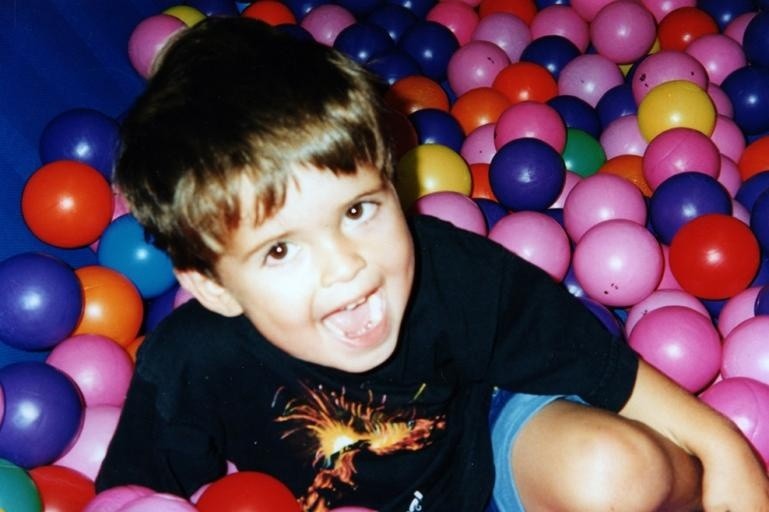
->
<box><xmin>95</xmin><ymin>12</ymin><xmax>769</xmax><ymax>511</ymax></box>
